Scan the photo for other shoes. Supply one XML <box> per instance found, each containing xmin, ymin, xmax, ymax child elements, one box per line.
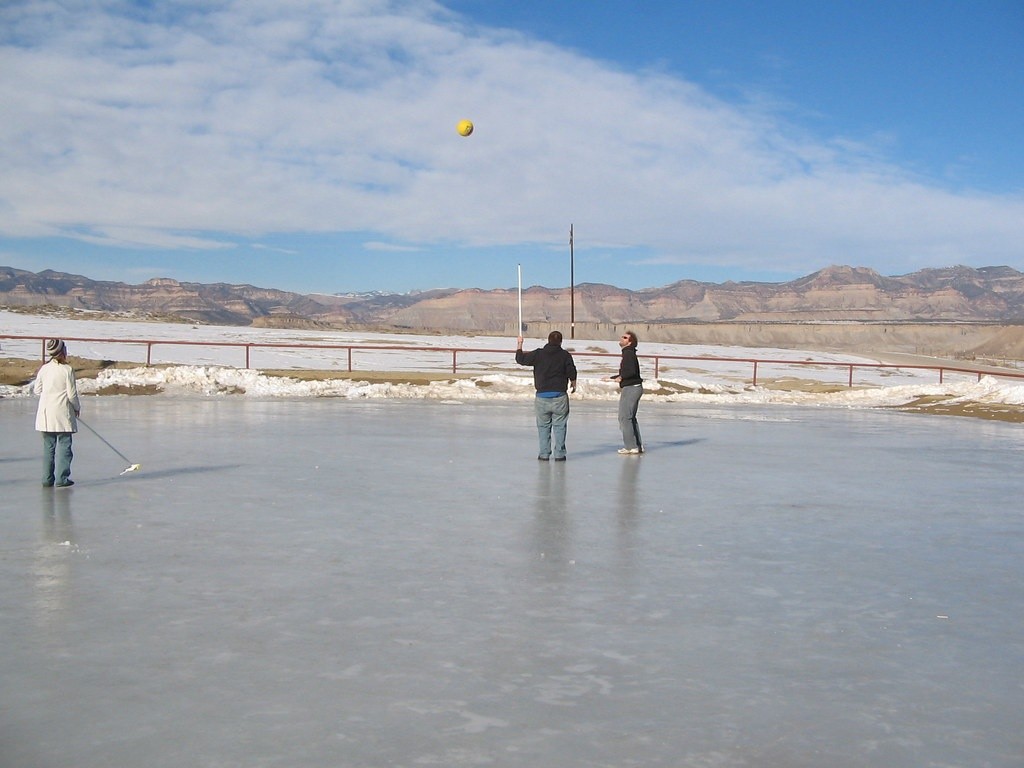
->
<box><xmin>43</xmin><ymin>484</ymin><xmax>54</xmax><ymax>487</ymax></box>
<box><xmin>555</xmin><ymin>455</ymin><xmax>566</xmax><ymax>461</ymax></box>
<box><xmin>538</xmin><ymin>455</ymin><xmax>549</xmax><ymax>461</ymax></box>
<box><xmin>57</xmin><ymin>479</ymin><xmax>75</xmax><ymax>489</ymax></box>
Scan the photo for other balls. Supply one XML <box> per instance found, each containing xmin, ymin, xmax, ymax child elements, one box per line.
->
<box><xmin>457</xmin><ymin>119</ymin><xmax>474</xmax><ymax>137</ymax></box>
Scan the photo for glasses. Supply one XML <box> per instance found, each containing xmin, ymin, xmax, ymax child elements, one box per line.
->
<box><xmin>622</xmin><ymin>336</ymin><xmax>630</xmax><ymax>340</ymax></box>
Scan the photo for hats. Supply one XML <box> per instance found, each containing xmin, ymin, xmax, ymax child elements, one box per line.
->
<box><xmin>46</xmin><ymin>339</ymin><xmax>65</xmax><ymax>358</ymax></box>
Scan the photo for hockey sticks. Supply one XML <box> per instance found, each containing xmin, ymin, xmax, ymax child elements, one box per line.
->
<box><xmin>75</xmin><ymin>417</ymin><xmax>140</xmax><ymax>476</ymax></box>
<box><xmin>518</xmin><ymin>263</ymin><xmax>523</xmax><ymax>337</ymax></box>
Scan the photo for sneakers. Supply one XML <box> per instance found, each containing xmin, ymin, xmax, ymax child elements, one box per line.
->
<box><xmin>639</xmin><ymin>446</ymin><xmax>643</xmax><ymax>453</ymax></box>
<box><xmin>618</xmin><ymin>448</ymin><xmax>639</xmax><ymax>454</ymax></box>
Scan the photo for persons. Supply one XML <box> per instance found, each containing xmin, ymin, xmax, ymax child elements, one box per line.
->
<box><xmin>33</xmin><ymin>338</ymin><xmax>81</xmax><ymax>488</ymax></box>
<box><xmin>601</xmin><ymin>331</ymin><xmax>645</xmax><ymax>454</ymax></box>
<box><xmin>515</xmin><ymin>331</ymin><xmax>577</xmax><ymax>461</ymax></box>
